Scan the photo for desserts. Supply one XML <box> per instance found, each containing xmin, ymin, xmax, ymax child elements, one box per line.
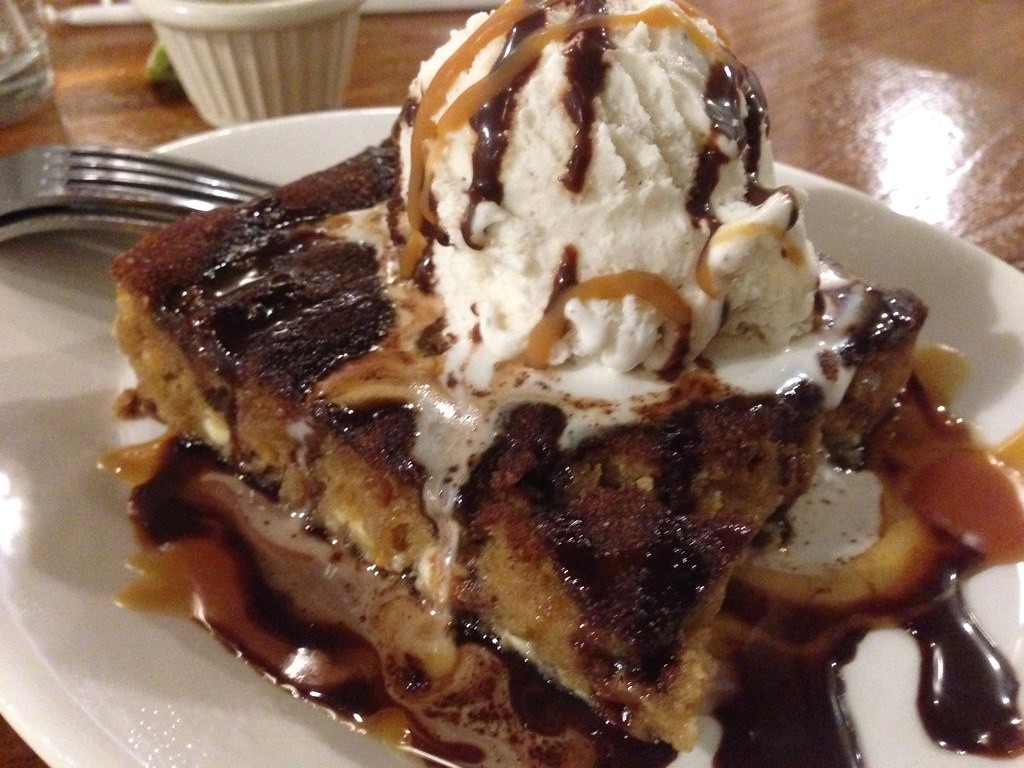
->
<box><xmin>105</xmin><ymin>0</ymin><xmax>1024</xmax><ymax>768</ymax></box>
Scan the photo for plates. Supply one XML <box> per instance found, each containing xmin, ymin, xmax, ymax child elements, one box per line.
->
<box><xmin>0</xmin><ymin>108</ymin><xmax>1024</xmax><ymax>767</ymax></box>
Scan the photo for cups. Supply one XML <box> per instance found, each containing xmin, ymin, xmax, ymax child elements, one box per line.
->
<box><xmin>0</xmin><ymin>1</ymin><xmax>56</xmax><ymax>123</ymax></box>
<box><xmin>135</xmin><ymin>0</ymin><xmax>364</xmax><ymax>130</ymax></box>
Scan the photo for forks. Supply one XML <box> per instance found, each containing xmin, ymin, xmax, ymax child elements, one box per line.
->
<box><xmin>0</xmin><ymin>144</ymin><xmax>279</xmax><ymax>217</ymax></box>
